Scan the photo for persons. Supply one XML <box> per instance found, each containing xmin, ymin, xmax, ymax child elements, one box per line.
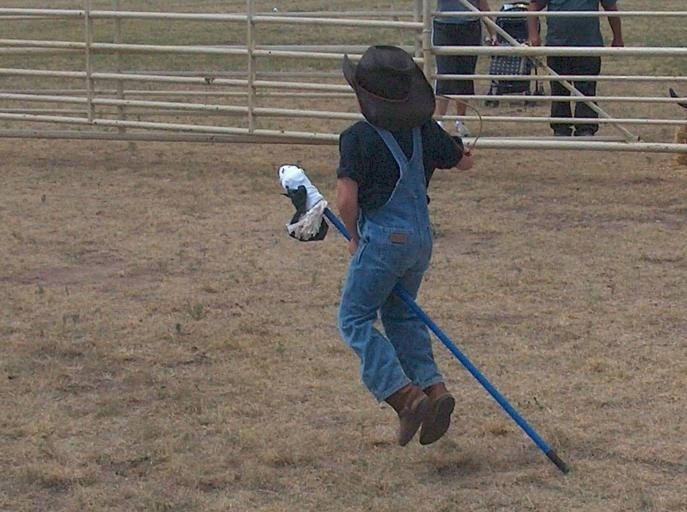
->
<box><xmin>337</xmin><ymin>44</ymin><xmax>475</xmax><ymax>449</ymax></box>
<box><xmin>430</xmin><ymin>1</ymin><xmax>498</xmax><ymax>139</ymax></box>
<box><xmin>527</xmin><ymin>1</ymin><xmax>623</xmax><ymax>137</ymax></box>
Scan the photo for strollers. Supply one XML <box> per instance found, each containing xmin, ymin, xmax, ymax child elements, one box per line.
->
<box><xmin>485</xmin><ymin>2</ymin><xmax>545</xmax><ymax>108</ymax></box>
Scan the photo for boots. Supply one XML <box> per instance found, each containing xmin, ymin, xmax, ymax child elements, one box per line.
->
<box><xmin>384</xmin><ymin>381</ymin><xmax>431</xmax><ymax>447</ymax></box>
<box><xmin>419</xmin><ymin>381</ymin><xmax>455</xmax><ymax>444</ymax></box>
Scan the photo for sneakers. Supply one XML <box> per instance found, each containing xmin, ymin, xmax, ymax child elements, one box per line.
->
<box><xmin>455</xmin><ymin>120</ymin><xmax>471</xmax><ymax>137</ymax></box>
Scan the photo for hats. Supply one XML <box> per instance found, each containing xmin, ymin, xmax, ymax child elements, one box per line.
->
<box><xmin>342</xmin><ymin>40</ymin><xmax>437</xmax><ymax>133</ymax></box>
